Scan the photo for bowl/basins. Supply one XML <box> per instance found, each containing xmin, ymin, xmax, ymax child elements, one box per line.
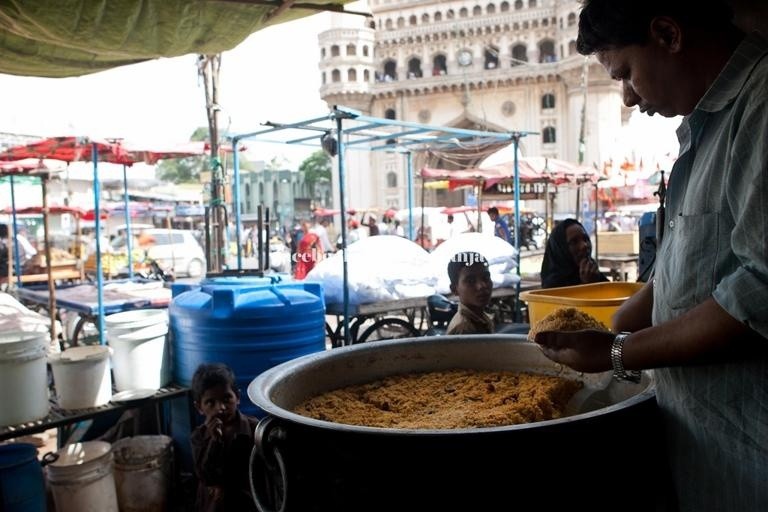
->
<box><xmin>246</xmin><ymin>333</ymin><xmax>657</xmax><ymax>510</ymax></box>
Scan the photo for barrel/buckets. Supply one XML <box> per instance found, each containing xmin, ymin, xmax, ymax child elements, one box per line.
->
<box><xmin>103</xmin><ymin>306</ymin><xmax>175</xmax><ymax>396</ymax></box>
<box><xmin>109</xmin><ymin>434</ymin><xmax>177</xmax><ymax>512</ymax></box>
<box><xmin>43</xmin><ymin>439</ymin><xmax>120</xmax><ymax>511</ymax></box>
<box><xmin>166</xmin><ymin>282</ymin><xmax>325</xmax><ymax>419</ymax></box>
<box><xmin>0</xmin><ymin>329</ymin><xmax>54</xmax><ymax>427</ymax></box>
<box><xmin>47</xmin><ymin>343</ymin><xmax>114</xmax><ymax>411</ymax></box>
<box><xmin>1</xmin><ymin>441</ymin><xmax>48</xmax><ymax>512</ymax></box>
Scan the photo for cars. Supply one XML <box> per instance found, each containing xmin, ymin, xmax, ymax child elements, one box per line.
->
<box><xmin>100</xmin><ymin>227</ymin><xmax>205</xmax><ymax>283</ymax></box>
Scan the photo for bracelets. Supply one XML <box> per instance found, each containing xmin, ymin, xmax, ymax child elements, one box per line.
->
<box><xmin>611</xmin><ymin>331</ymin><xmax>643</xmax><ymax>388</ymax></box>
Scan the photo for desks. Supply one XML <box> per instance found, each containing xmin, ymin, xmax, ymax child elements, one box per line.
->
<box><xmin>0</xmin><ymin>379</ymin><xmax>199</xmax><ymax>463</ymax></box>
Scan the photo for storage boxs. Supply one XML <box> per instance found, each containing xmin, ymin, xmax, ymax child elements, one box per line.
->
<box><xmin>596</xmin><ymin>231</ymin><xmax>641</xmax><ymax>258</ymax></box>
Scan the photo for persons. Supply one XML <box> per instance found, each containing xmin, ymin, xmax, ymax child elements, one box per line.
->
<box><xmin>534</xmin><ymin>1</ymin><xmax>768</xmax><ymax>511</ymax></box>
<box><xmin>444</xmin><ymin>250</ymin><xmax>500</xmax><ymax>337</ymax></box>
<box><xmin>227</xmin><ymin>206</ymin><xmax>513</xmax><ymax>281</ymax></box>
<box><xmin>189</xmin><ymin>360</ymin><xmax>266</xmax><ymax>510</ymax></box>
<box><xmin>1</xmin><ymin>222</ymin><xmax>12</xmax><ymax>295</ymax></box>
<box><xmin>540</xmin><ymin>218</ymin><xmax>610</xmax><ymax>289</ymax></box>
